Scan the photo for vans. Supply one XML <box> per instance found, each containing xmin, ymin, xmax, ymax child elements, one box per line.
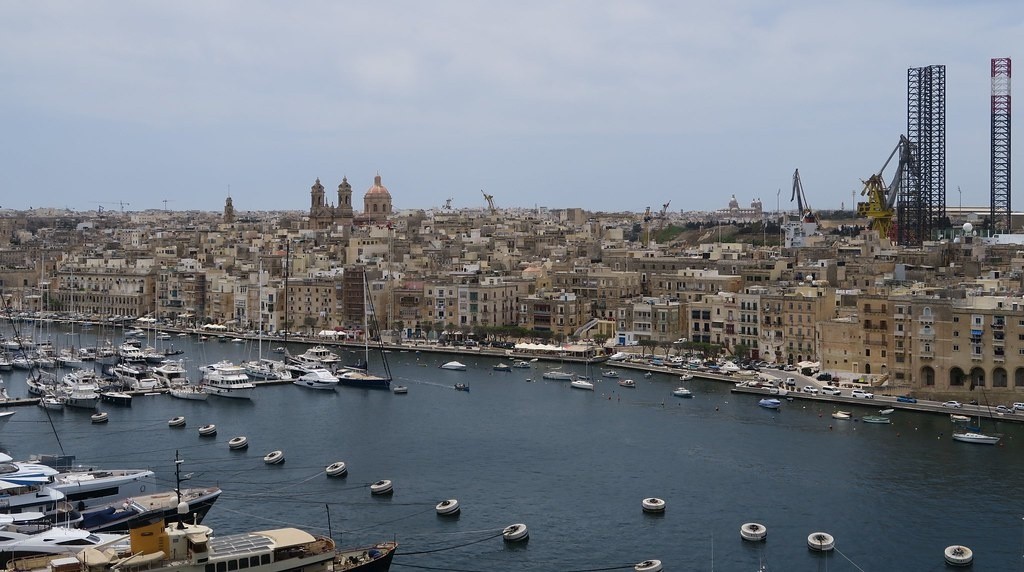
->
<box><xmin>786</xmin><ymin>378</ymin><xmax>795</xmax><ymax>385</ymax></box>
<box><xmin>821</xmin><ymin>386</ymin><xmax>842</xmax><ymax>395</ymax></box>
<box><xmin>674</xmin><ymin>338</ymin><xmax>687</xmax><ymax>343</ymax></box>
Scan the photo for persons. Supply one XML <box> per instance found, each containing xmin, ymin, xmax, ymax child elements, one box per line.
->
<box><xmin>369</xmin><ymin>547</ymin><xmax>380</xmax><ymax>558</ymax></box>
<box><xmin>348</xmin><ymin>556</ymin><xmax>359</xmax><ymax>565</ymax></box>
<box><xmin>362</xmin><ymin>551</ymin><xmax>370</xmax><ymax>561</ymax></box>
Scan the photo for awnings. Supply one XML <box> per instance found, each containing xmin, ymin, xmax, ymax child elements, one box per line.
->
<box><xmin>319</xmin><ymin>330</ymin><xmax>337</xmax><ymax>337</ymax></box>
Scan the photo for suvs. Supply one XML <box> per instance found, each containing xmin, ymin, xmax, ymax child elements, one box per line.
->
<box><xmin>852</xmin><ymin>389</ymin><xmax>873</xmax><ymax>399</ymax></box>
<box><xmin>1013</xmin><ymin>402</ymin><xmax>1024</xmax><ymax>410</ymax></box>
<box><xmin>995</xmin><ymin>406</ymin><xmax>1015</xmax><ymax>414</ymax></box>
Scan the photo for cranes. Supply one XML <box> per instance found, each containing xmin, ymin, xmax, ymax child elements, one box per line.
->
<box><xmin>90</xmin><ymin>200</ymin><xmax>130</xmax><ymax>212</ymax></box>
<box><xmin>863</xmin><ymin>134</ymin><xmax>918</xmax><ymax>242</ymax></box>
<box><xmin>161</xmin><ymin>197</ymin><xmax>174</xmax><ymax>209</ymax></box>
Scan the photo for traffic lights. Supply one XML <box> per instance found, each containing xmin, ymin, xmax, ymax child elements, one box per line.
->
<box><xmin>862</xmin><ymin>415</ymin><xmax>891</xmax><ymax>424</ymax></box>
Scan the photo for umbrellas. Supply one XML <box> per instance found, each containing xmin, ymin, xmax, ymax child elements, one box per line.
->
<box><xmin>511</xmin><ymin>343</ymin><xmax>565</xmax><ymax>355</ymax></box>
<box><xmin>336</xmin><ymin>331</ymin><xmax>348</xmax><ymax>336</ymax></box>
<box><xmin>137</xmin><ymin>317</ymin><xmax>157</xmax><ymax>326</ymax></box>
<box><xmin>442</xmin><ymin>330</ymin><xmax>474</xmax><ymax>341</ymax></box>
<box><xmin>204</xmin><ymin>324</ymin><xmax>227</xmax><ymax>331</ymax></box>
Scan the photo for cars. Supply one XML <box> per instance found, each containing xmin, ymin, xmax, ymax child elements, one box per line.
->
<box><xmin>895</xmin><ymin>395</ymin><xmax>918</xmax><ymax>403</ymax></box>
<box><xmin>803</xmin><ymin>386</ymin><xmax>818</xmax><ymax>392</ymax></box>
<box><xmin>942</xmin><ymin>401</ymin><xmax>964</xmax><ymax>409</ymax></box>
<box><xmin>732</xmin><ymin>357</ymin><xmax>798</xmax><ymax>371</ymax></box>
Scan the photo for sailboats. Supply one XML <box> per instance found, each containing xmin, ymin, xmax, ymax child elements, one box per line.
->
<box><xmin>546</xmin><ymin>327</ymin><xmax>574</xmax><ymax>380</ymax></box>
<box><xmin>0</xmin><ymin>242</ymin><xmax>394</xmax><ymax>410</ymax></box>
<box><xmin>571</xmin><ymin>328</ymin><xmax>594</xmax><ymax>392</ymax></box>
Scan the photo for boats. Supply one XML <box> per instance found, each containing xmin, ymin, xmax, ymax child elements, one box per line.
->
<box><xmin>760</xmin><ymin>399</ymin><xmax>781</xmax><ymax>410</ymax></box>
<box><xmin>618</xmin><ymin>379</ymin><xmax>636</xmax><ymax>388</ymax></box>
<box><xmin>0</xmin><ymin>451</ymin><xmax>398</xmax><ymax>572</ymax></box>
<box><xmin>591</xmin><ymin>350</ymin><xmax>740</xmax><ymax>374</ymax></box>
<box><xmin>832</xmin><ymin>411</ymin><xmax>852</xmax><ymax>420</ymax></box>
<box><xmin>442</xmin><ymin>360</ymin><xmax>466</xmax><ymax>371</ymax></box>
<box><xmin>673</xmin><ymin>386</ymin><xmax>692</xmax><ymax>398</ymax></box>
<box><xmin>601</xmin><ymin>369</ymin><xmax>619</xmax><ymax>377</ymax></box>
<box><xmin>493</xmin><ymin>363</ymin><xmax>511</xmax><ymax>371</ymax></box>
<box><xmin>514</xmin><ymin>360</ymin><xmax>530</xmax><ymax>368</ymax></box>
